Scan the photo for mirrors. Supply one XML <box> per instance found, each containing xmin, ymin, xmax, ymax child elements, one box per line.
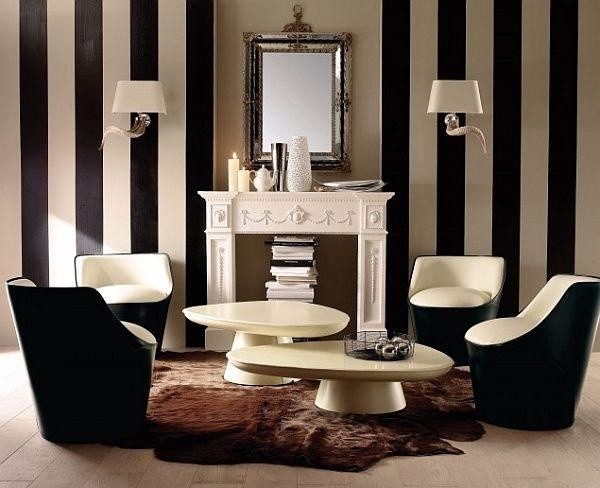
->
<box><xmin>242</xmin><ymin>29</ymin><xmax>353</xmax><ymax>174</ymax></box>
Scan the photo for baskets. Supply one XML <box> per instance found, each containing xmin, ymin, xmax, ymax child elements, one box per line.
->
<box><xmin>343</xmin><ymin>330</ymin><xmax>416</xmax><ymax>360</ymax></box>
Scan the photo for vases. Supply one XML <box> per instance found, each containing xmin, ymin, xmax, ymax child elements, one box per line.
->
<box><xmin>285</xmin><ymin>134</ymin><xmax>313</xmax><ymax>192</ymax></box>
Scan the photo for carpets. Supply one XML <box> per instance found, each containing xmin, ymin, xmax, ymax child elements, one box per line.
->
<box><xmin>147</xmin><ymin>341</ymin><xmax>485</xmax><ymax>473</ymax></box>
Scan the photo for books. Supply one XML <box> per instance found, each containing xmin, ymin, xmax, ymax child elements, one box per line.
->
<box><xmin>260</xmin><ymin>231</ymin><xmax>320</xmax><ymax>305</ymax></box>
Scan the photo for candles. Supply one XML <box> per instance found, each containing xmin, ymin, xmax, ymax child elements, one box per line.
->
<box><xmin>237</xmin><ymin>166</ymin><xmax>250</xmax><ymax>192</ymax></box>
<box><xmin>227</xmin><ymin>152</ymin><xmax>239</xmax><ymax>191</ymax></box>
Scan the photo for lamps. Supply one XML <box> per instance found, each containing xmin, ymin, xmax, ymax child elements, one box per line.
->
<box><xmin>425</xmin><ymin>78</ymin><xmax>489</xmax><ymax>158</ymax></box>
<box><xmin>95</xmin><ymin>79</ymin><xmax>169</xmax><ymax>150</ymax></box>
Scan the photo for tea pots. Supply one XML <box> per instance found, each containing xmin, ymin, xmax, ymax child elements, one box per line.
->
<box><xmin>249</xmin><ymin>165</ymin><xmax>279</xmax><ymax>192</ymax></box>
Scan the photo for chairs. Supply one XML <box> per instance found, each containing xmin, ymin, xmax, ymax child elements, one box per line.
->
<box><xmin>407</xmin><ymin>253</ymin><xmax>506</xmax><ymax>364</ymax></box>
<box><xmin>3</xmin><ymin>275</ymin><xmax>158</xmax><ymax>446</ymax></box>
<box><xmin>464</xmin><ymin>272</ymin><xmax>600</xmax><ymax>430</ymax></box>
<box><xmin>69</xmin><ymin>252</ymin><xmax>175</xmax><ymax>361</ymax></box>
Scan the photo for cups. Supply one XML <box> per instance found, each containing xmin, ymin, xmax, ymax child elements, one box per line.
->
<box><xmin>270</xmin><ymin>142</ymin><xmax>288</xmax><ymax>190</ymax></box>
